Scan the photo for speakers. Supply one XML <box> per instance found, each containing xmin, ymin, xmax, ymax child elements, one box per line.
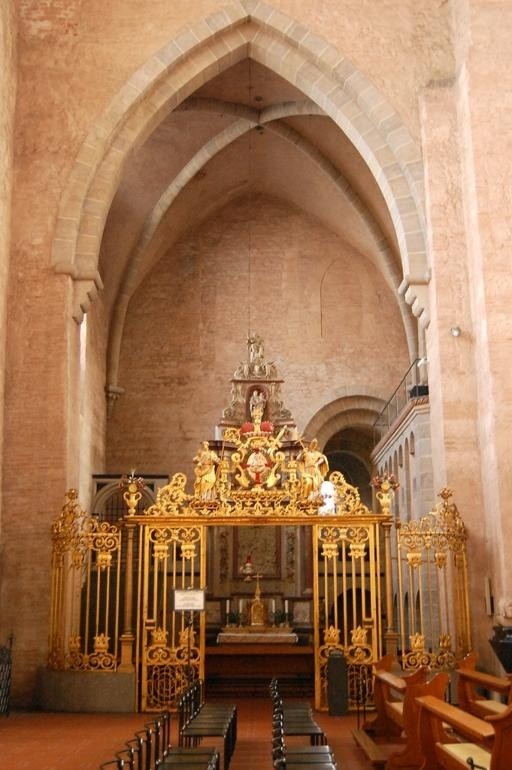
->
<box><xmin>409</xmin><ymin>385</ymin><xmax>428</xmax><ymax>398</ymax></box>
<box><xmin>327</xmin><ymin>655</ymin><xmax>347</xmax><ymax>716</ymax></box>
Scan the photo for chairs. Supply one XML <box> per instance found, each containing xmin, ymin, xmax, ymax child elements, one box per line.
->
<box><xmin>269</xmin><ymin>674</ymin><xmax>338</xmax><ymax>770</ymax></box>
<box><xmin>101</xmin><ymin>680</ymin><xmax>240</xmax><ymax>770</ymax></box>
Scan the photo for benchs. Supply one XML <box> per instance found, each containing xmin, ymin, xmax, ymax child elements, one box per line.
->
<box><xmin>349</xmin><ymin>653</ymin><xmax>511</xmax><ymax>770</ymax></box>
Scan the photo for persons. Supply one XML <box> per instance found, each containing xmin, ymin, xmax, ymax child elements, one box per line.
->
<box><xmin>191</xmin><ymin>331</ymin><xmax>329</xmax><ymax>494</ymax></box>
